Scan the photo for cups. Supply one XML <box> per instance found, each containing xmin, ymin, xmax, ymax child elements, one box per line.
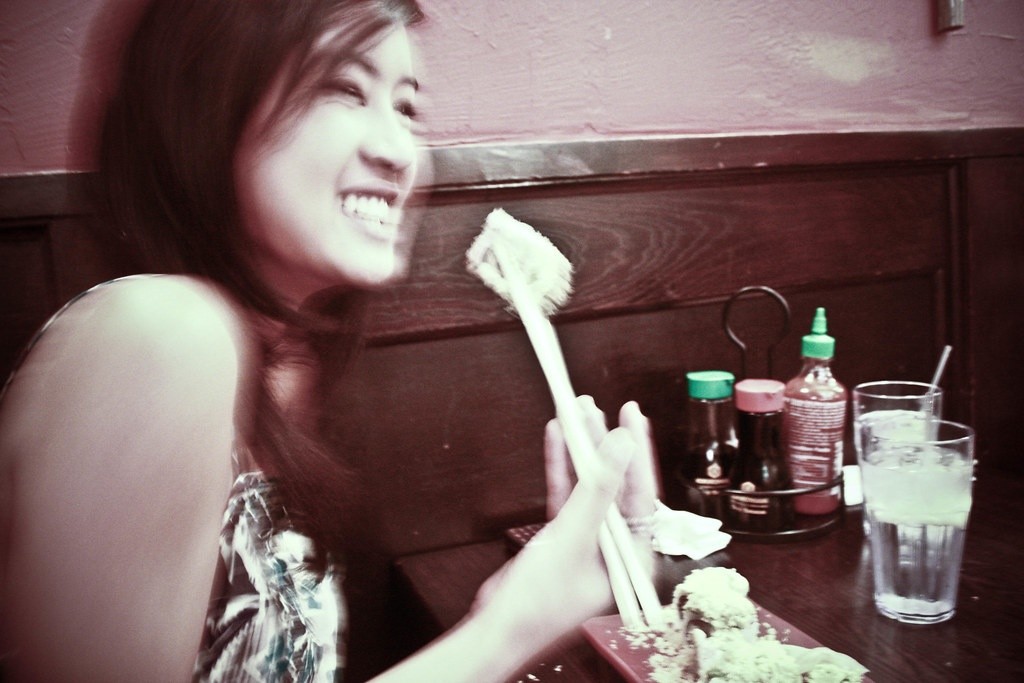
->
<box><xmin>862</xmin><ymin>414</ymin><xmax>975</xmax><ymax>625</ymax></box>
<box><xmin>852</xmin><ymin>379</ymin><xmax>944</xmax><ymax>465</ymax></box>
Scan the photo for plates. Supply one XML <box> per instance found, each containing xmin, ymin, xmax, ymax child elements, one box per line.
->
<box><xmin>511</xmin><ymin>520</ymin><xmax>873</xmax><ymax>683</ymax></box>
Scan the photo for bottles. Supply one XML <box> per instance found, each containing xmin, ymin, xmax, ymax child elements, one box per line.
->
<box><xmin>728</xmin><ymin>376</ymin><xmax>790</xmax><ymax>534</ymax></box>
<box><xmin>679</xmin><ymin>364</ymin><xmax>734</xmax><ymax>524</ymax></box>
<box><xmin>778</xmin><ymin>306</ymin><xmax>847</xmax><ymax>517</ymax></box>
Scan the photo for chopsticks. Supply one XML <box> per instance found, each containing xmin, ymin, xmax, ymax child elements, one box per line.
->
<box><xmin>490</xmin><ymin>244</ymin><xmax>667</xmax><ymax>637</ymax></box>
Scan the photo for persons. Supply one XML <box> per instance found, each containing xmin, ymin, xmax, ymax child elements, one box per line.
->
<box><xmin>0</xmin><ymin>2</ymin><xmax>660</xmax><ymax>683</ymax></box>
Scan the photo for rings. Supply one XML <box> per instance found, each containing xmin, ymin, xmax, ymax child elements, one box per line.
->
<box><xmin>625</xmin><ymin>517</ymin><xmax>657</xmax><ymax>535</ymax></box>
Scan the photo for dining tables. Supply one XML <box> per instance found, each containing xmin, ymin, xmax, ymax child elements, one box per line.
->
<box><xmin>388</xmin><ymin>445</ymin><xmax>1024</xmax><ymax>683</ymax></box>
